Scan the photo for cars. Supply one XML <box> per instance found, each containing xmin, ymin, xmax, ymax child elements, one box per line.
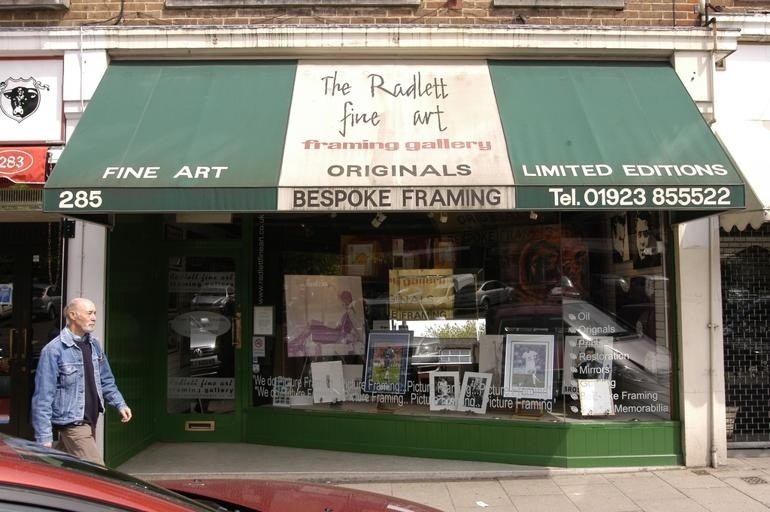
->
<box><xmin>726</xmin><ymin>298</ymin><xmax>770</xmax><ymax>375</ymax></box>
<box><xmin>3</xmin><ymin>430</ymin><xmax>450</xmax><ymax>512</ymax></box>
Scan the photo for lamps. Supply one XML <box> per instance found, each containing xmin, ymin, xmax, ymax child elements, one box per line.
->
<box><xmin>368</xmin><ymin>210</ymin><xmax>539</xmax><ymax>229</ymax></box>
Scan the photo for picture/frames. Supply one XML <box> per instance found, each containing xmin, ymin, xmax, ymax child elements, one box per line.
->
<box><xmin>359</xmin><ymin>330</ymin><xmax>617</xmax><ymax>418</ymax></box>
<box><xmin>341</xmin><ymin>237</ymin><xmax>457</xmax><ymax>280</ymax></box>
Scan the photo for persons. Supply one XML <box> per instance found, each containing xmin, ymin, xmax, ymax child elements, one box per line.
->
<box><xmin>289</xmin><ymin>289</ymin><xmax>366</xmax><ymax>354</ymax></box>
<box><xmin>632</xmin><ymin>212</ymin><xmax>662</xmax><ymax>270</ymax></box>
<box><xmin>29</xmin><ymin>297</ymin><xmax>134</xmax><ymax>467</ymax></box>
<box><xmin>610</xmin><ymin>215</ymin><xmax>626</xmax><ymax>264</ymax></box>
<box><xmin>440</xmin><ymin>378</ymin><xmax>450</xmax><ymax>395</ymax></box>
<box><xmin>382</xmin><ymin>346</ymin><xmax>396</xmax><ymax>383</ymax></box>
<box><xmin>520</xmin><ymin>347</ymin><xmax>541</xmax><ymax>387</ymax></box>
<box><xmin>572</xmin><ymin>346</ymin><xmax>602</xmax><ymax>378</ymax></box>
<box><xmin>604</xmin><ymin>364</ymin><xmax>612</xmax><ymax>379</ymax></box>
